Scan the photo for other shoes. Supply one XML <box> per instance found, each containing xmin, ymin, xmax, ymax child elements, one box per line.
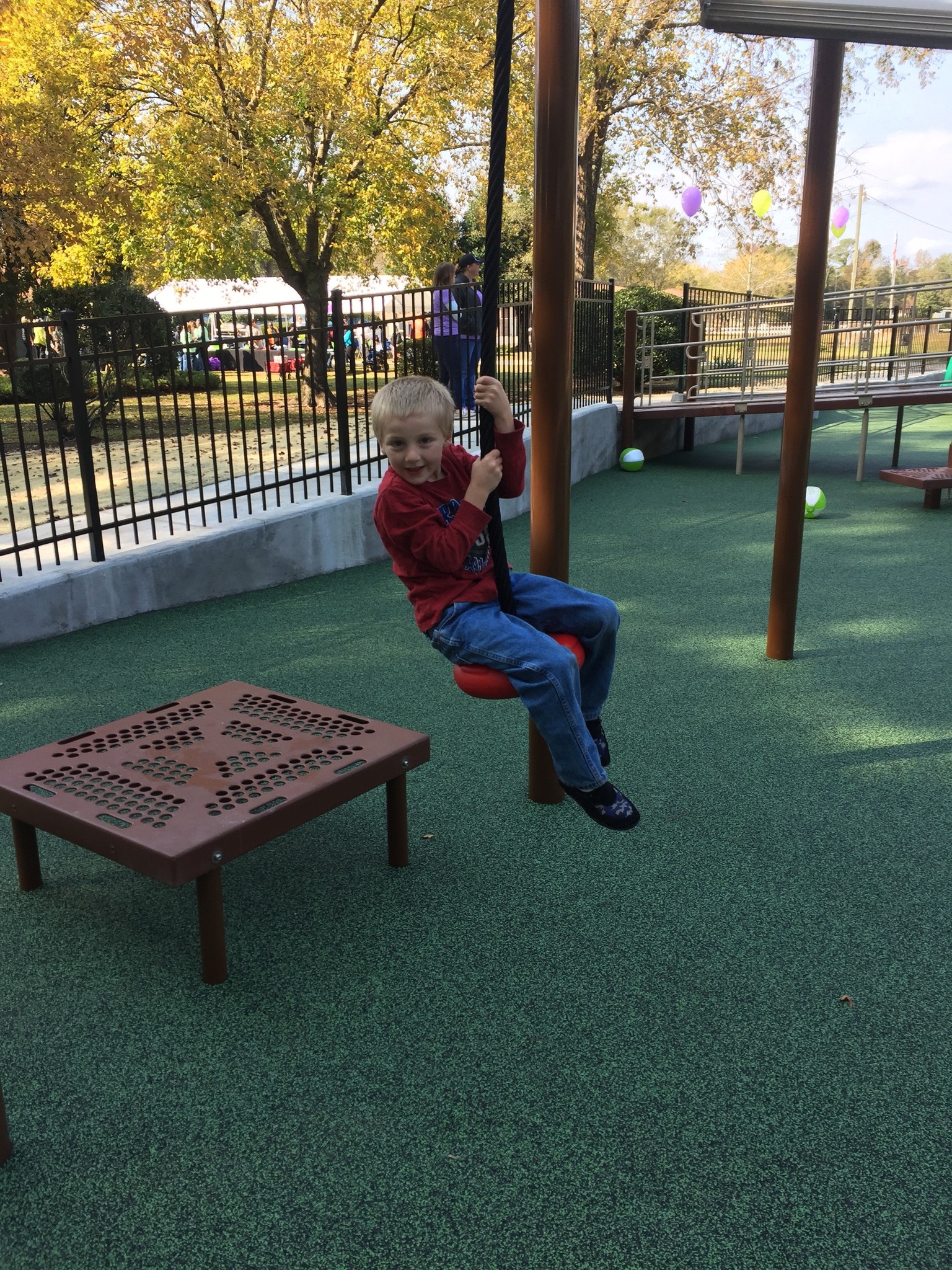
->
<box><xmin>473</xmin><ymin>406</ymin><xmax>480</xmax><ymax>412</ymax></box>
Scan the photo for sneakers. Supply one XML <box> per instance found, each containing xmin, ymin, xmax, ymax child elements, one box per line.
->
<box><xmin>453</xmin><ymin>409</ymin><xmax>476</xmax><ymax>420</ymax></box>
<box><xmin>558</xmin><ymin>781</ymin><xmax>641</xmax><ymax>831</ymax></box>
<box><xmin>586</xmin><ymin>717</ymin><xmax>610</xmax><ymax>766</ymax></box>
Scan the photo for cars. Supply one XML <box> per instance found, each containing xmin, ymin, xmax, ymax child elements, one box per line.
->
<box><xmin>346</xmin><ymin>317</ymin><xmax>371</xmax><ymax>325</ymax></box>
<box><xmin>215</xmin><ymin>322</ymin><xmax>246</xmax><ymax>347</ymax></box>
<box><xmin>259</xmin><ymin>317</ymin><xmax>293</xmax><ymax>335</ymax></box>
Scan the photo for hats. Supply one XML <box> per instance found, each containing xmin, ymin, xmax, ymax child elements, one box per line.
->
<box><xmin>459</xmin><ymin>254</ymin><xmax>481</xmax><ymax>267</ymax></box>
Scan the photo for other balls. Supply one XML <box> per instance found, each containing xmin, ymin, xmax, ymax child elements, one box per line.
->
<box><xmin>619</xmin><ymin>447</ymin><xmax>645</xmax><ymax>472</ymax></box>
<box><xmin>800</xmin><ymin>486</ymin><xmax>827</xmax><ymax>519</ymax></box>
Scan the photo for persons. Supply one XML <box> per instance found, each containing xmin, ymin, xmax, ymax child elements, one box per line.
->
<box><xmin>343</xmin><ymin>323</ymin><xmax>352</xmax><ymax>373</ymax></box>
<box><xmin>173</xmin><ymin>319</ymin><xmax>208</xmax><ymax>371</ymax></box>
<box><xmin>425</xmin><ymin>317</ymin><xmax>432</xmax><ymax>338</ymax></box>
<box><xmin>409</xmin><ymin>313</ymin><xmax>427</xmax><ymax>340</ymax></box>
<box><xmin>355</xmin><ymin>319</ymin><xmax>370</xmax><ymax>363</ymax></box>
<box><xmin>267</xmin><ymin>322</ymin><xmax>280</xmax><ymax>350</ymax></box>
<box><xmin>245</xmin><ymin>319</ymin><xmax>263</xmax><ymax>347</ymax></box>
<box><xmin>432</xmin><ymin>261</ymin><xmax>476</xmax><ymax>419</ymax></box>
<box><xmin>451</xmin><ymin>253</ymin><xmax>484</xmax><ymax>413</ymax></box>
<box><xmin>287</xmin><ymin>323</ymin><xmax>301</xmax><ymax>348</ymax></box>
<box><xmin>327</xmin><ymin>330</ymin><xmax>334</xmax><ymax>368</ymax></box>
<box><xmin>21</xmin><ymin>316</ymin><xmax>60</xmax><ymax>358</ymax></box>
<box><xmin>390</xmin><ymin>325</ymin><xmax>401</xmax><ymax>362</ymax></box>
<box><xmin>371</xmin><ymin>375</ymin><xmax>640</xmax><ymax>831</ymax></box>
<box><xmin>375</xmin><ymin>337</ymin><xmax>384</xmax><ymax>352</ymax></box>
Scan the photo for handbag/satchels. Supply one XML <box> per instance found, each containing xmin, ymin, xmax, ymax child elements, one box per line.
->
<box><xmin>208</xmin><ymin>357</ymin><xmax>222</xmax><ymax>371</ymax></box>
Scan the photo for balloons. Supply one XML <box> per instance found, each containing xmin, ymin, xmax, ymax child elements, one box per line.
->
<box><xmin>831</xmin><ymin>222</ymin><xmax>846</xmax><ymax>238</ymax></box>
<box><xmin>681</xmin><ymin>186</ymin><xmax>702</xmax><ymax>217</ymax></box>
<box><xmin>298</xmin><ymin>334</ymin><xmax>305</xmax><ymax>340</ymax></box>
<box><xmin>833</xmin><ymin>206</ymin><xmax>849</xmax><ymax>228</ymax></box>
<box><xmin>752</xmin><ymin>190</ymin><xmax>771</xmax><ymax>217</ymax></box>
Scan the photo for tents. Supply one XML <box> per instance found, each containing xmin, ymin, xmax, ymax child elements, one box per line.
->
<box><xmin>146</xmin><ymin>276</ymin><xmax>431</xmax><ymax>327</ymax></box>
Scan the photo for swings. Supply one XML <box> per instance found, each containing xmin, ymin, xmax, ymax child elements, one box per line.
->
<box><xmin>452</xmin><ymin>0</ymin><xmax>586</xmax><ymax>700</ymax></box>
